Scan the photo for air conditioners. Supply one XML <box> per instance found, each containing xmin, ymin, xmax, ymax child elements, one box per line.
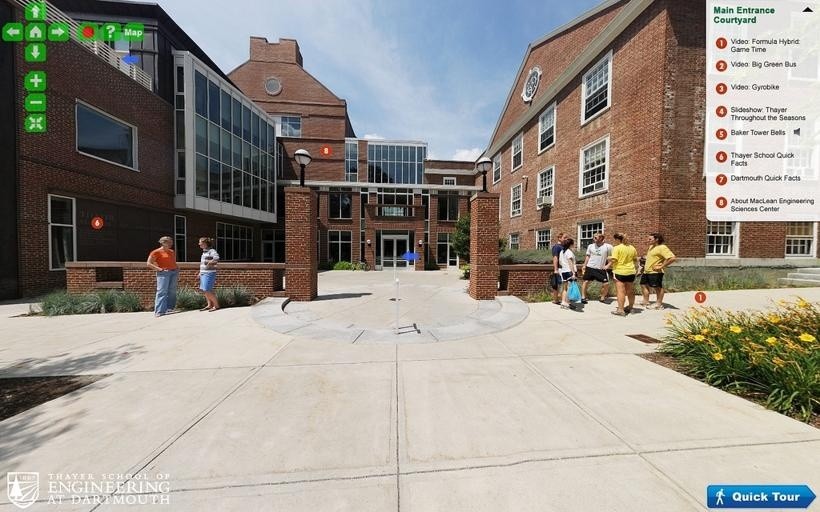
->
<box><xmin>536</xmin><ymin>196</ymin><xmax>551</xmax><ymax>205</ymax></box>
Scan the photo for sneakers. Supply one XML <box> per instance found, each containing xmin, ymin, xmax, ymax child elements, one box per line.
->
<box><xmin>611</xmin><ymin>311</ymin><xmax>626</xmax><ymax>316</ymax></box>
<box><xmin>209</xmin><ymin>305</ymin><xmax>221</xmax><ymax>311</ymax></box>
<box><xmin>561</xmin><ymin>303</ymin><xmax>576</xmax><ymax>310</ymax></box>
<box><xmin>200</xmin><ymin>306</ymin><xmax>214</xmax><ymax>311</ymax></box>
<box><xmin>646</xmin><ymin>302</ymin><xmax>665</xmax><ymax>310</ymax></box>
<box><xmin>624</xmin><ymin>306</ymin><xmax>634</xmax><ymax>313</ymax></box>
<box><xmin>581</xmin><ymin>298</ymin><xmax>586</xmax><ymax>303</ymax></box>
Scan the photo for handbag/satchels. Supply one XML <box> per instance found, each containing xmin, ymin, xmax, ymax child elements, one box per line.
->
<box><xmin>204</xmin><ymin>252</ymin><xmax>217</xmax><ymax>265</ymax></box>
<box><xmin>567</xmin><ymin>280</ymin><xmax>581</xmax><ymax>302</ymax></box>
<box><xmin>550</xmin><ymin>273</ymin><xmax>562</xmax><ymax>290</ymax></box>
